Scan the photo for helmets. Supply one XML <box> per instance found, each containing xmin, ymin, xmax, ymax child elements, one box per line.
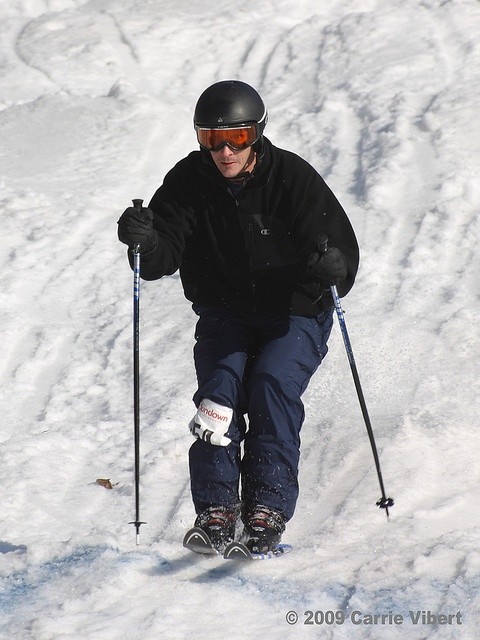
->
<box><xmin>194</xmin><ymin>80</ymin><xmax>268</xmax><ymax>135</ymax></box>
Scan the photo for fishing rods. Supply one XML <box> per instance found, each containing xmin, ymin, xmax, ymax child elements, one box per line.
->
<box><xmin>183</xmin><ymin>528</ymin><xmax>292</xmax><ymax>560</ymax></box>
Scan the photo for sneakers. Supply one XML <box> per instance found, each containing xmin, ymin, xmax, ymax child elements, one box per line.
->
<box><xmin>193</xmin><ymin>505</ymin><xmax>240</xmax><ymax>550</ymax></box>
<box><xmin>238</xmin><ymin>505</ymin><xmax>285</xmax><ymax>554</ymax></box>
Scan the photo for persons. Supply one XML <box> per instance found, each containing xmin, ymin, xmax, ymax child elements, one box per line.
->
<box><xmin>117</xmin><ymin>81</ymin><xmax>359</xmax><ymax>553</ymax></box>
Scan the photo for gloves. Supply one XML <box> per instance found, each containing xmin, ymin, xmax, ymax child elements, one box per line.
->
<box><xmin>308</xmin><ymin>247</ymin><xmax>347</xmax><ymax>292</ymax></box>
<box><xmin>117</xmin><ymin>207</ymin><xmax>160</xmax><ymax>258</ymax></box>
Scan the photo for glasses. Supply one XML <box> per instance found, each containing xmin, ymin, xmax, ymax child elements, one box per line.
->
<box><xmin>195</xmin><ymin>114</ymin><xmax>268</xmax><ymax>152</ymax></box>
